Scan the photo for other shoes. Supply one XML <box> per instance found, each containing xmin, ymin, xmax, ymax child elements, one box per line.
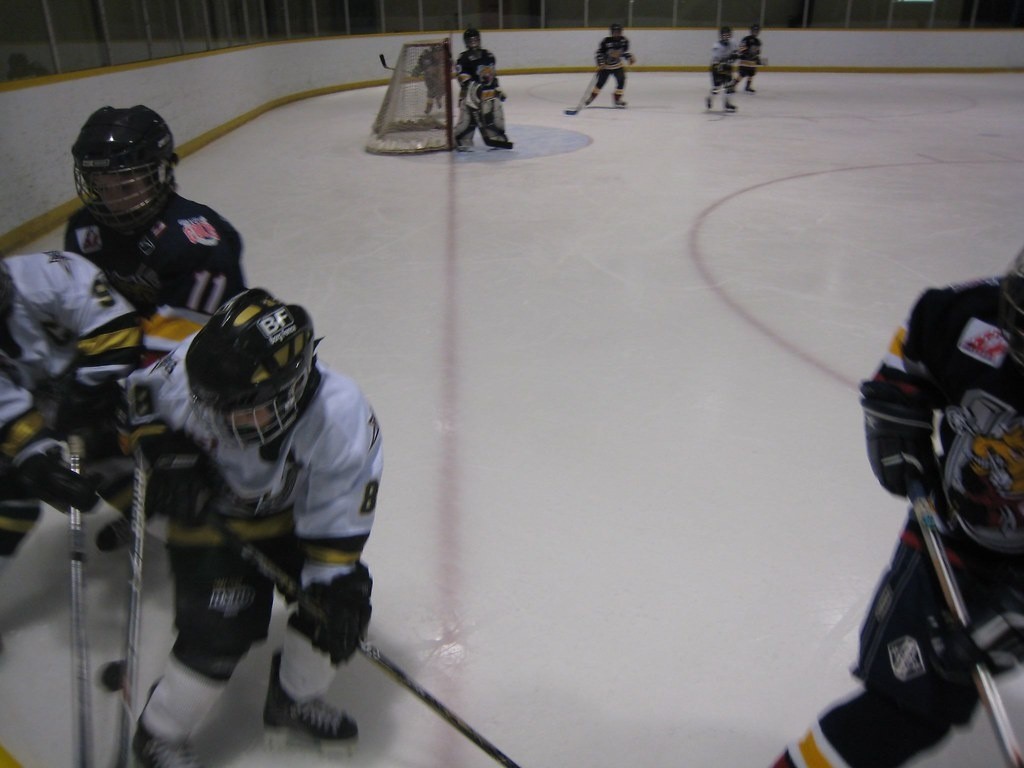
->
<box><xmin>424</xmin><ymin>103</ymin><xmax>433</xmax><ymax>113</ymax></box>
<box><xmin>436</xmin><ymin>99</ymin><xmax>442</xmax><ymax>109</ymax></box>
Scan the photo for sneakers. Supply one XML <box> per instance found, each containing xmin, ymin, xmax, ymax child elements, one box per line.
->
<box><xmin>613</xmin><ymin>94</ymin><xmax>626</xmax><ymax>106</ymax></box>
<box><xmin>95</xmin><ymin>516</ymin><xmax>140</xmax><ymax>554</ymax></box>
<box><xmin>263</xmin><ymin>649</ymin><xmax>359</xmax><ymax>741</ymax></box>
<box><xmin>132</xmin><ymin>677</ymin><xmax>206</xmax><ymax>768</ymax></box>
<box><xmin>586</xmin><ymin>92</ymin><xmax>598</xmax><ymax>104</ymax></box>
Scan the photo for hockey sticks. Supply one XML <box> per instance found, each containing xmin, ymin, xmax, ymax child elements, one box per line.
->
<box><xmin>203</xmin><ymin>513</ymin><xmax>520</xmax><ymax>768</ymax></box>
<box><xmin>907</xmin><ymin>477</ymin><xmax>1024</xmax><ymax>768</ymax></box>
<box><xmin>380</xmin><ymin>54</ymin><xmax>443</xmax><ymax>79</ymax></box>
<box><xmin>457</xmin><ymin>76</ymin><xmax>513</xmax><ymax>150</ymax></box>
<box><xmin>69</xmin><ymin>430</ymin><xmax>93</xmax><ymax>768</ymax></box>
<box><xmin>112</xmin><ymin>438</ymin><xmax>152</xmax><ymax>768</ymax></box>
<box><xmin>563</xmin><ymin>63</ymin><xmax>604</xmax><ymax>115</ymax></box>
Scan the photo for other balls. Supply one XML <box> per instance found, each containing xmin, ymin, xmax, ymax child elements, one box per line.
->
<box><xmin>98</xmin><ymin>661</ymin><xmax>130</xmax><ymax>691</ymax></box>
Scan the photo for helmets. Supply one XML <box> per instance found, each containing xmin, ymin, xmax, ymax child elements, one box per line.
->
<box><xmin>463</xmin><ymin>27</ymin><xmax>481</xmax><ymax>51</ymax></box>
<box><xmin>752</xmin><ymin>24</ymin><xmax>760</xmax><ymax>32</ymax></box>
<box><xmin>431</xmin><ymin>44</ymin><xmax>443</xmax><ymax>64</ymax></box>
<box><xmin>720</xmin><ymin>26</ymin><xmax>730</xmax><ymax>34</ymax></box>
<box><xmin>997</xmin><ymin>246</ymin><xmax>1024</xmax><ymax>368</ymax></box>
<box><xmin>70</xmin><ymin>105</ymin><xmax>180</xmax><ymax>240</ymax></box>
<box><xmin>186</xmin><ymin>286</ymin><xmax>326</xmax><ymax>456</ymax></box>
<box><xmin>610</xmin><ymin>23</ymin><xmax>623</xmax><ymax>32</ymax></box>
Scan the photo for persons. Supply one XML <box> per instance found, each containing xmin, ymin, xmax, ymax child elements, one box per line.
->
<box><xmin>410</xmin><ymin>44</ymin><xmax>458</xmax><ymax>113</ymax></box>
<box><xmin>768</xmin><ymin>247</ymin><xmax>1024</xmax><ymax>768</ymax></box>
<box><xmin>0</xmin><ymin>249</ymin><xmax>153</xmax><ymax>559</ymax></box>
<box><xmin>63</xmin><ymin>105</ymin><xmax>248</xmax><ymax>518</ymax></box>
<box><xmin>585</xmin><ymin>22</ymin><xmax>635</xmax><ymax>109</ymax></box>
<box><xmin>706</xmin><ymin>24</ymin><xmax>743</xmax><ymax>113</ymax></box>
<box><xmin>727</xmin><ymin>24</ymin><xmax>762</xmax><ymax>94</ymax></box>
<box><xmin>452</xmin><ymin>28</ymin><xmax>513</xmax><ymax>152</ymax></box>
<box><xmin>126</xmin><ymin>287</ymin><xmax>383</xmax><ymax>768</ymax></box>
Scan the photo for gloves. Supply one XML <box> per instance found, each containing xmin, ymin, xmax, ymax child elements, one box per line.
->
<box><xmin>137</xmin><ymin>430</ymin><xmax>219</xmax><ymax>528</ymax></box>
<box><xmin>39</xmin><ymin>370</ymin><xmax>105</xmax><ymax>440</ymax></box>
<box><xmin>730</xmin><ymin>53</ymin><xmax>738</xmax><ymax>60</ymax></box>
<box><xmin>411</xmin><ymin>68</ymin><xmax>421</xmax><ymax>77</ymax></box>
<box><xmin>923</xmin><ymin>579</ymin><xmax>1024</xmax><ymax>689</ymax></box>
<box><xmin>720</xmin><ymin>57</ymin><xmax>728</xmax><ymax>64</ymax></box>
<box><xmin>756</xmin><ymin>59</ymin><xmax>762</xmax><ymax>66</ymax></box>
<box><xmin>8</xmin><ymin>426</ymin><xmax>103</xmax><ymax>513</ymax></box>
<box><xmin>287</xmin><ymin>559</ymin><xmax>373</xmax><ymax>669</ymax></box>
<box><xmin>860</xmin><ymin>378</ymin><xmax>945</xmax><ymax>498</ymax></box>
